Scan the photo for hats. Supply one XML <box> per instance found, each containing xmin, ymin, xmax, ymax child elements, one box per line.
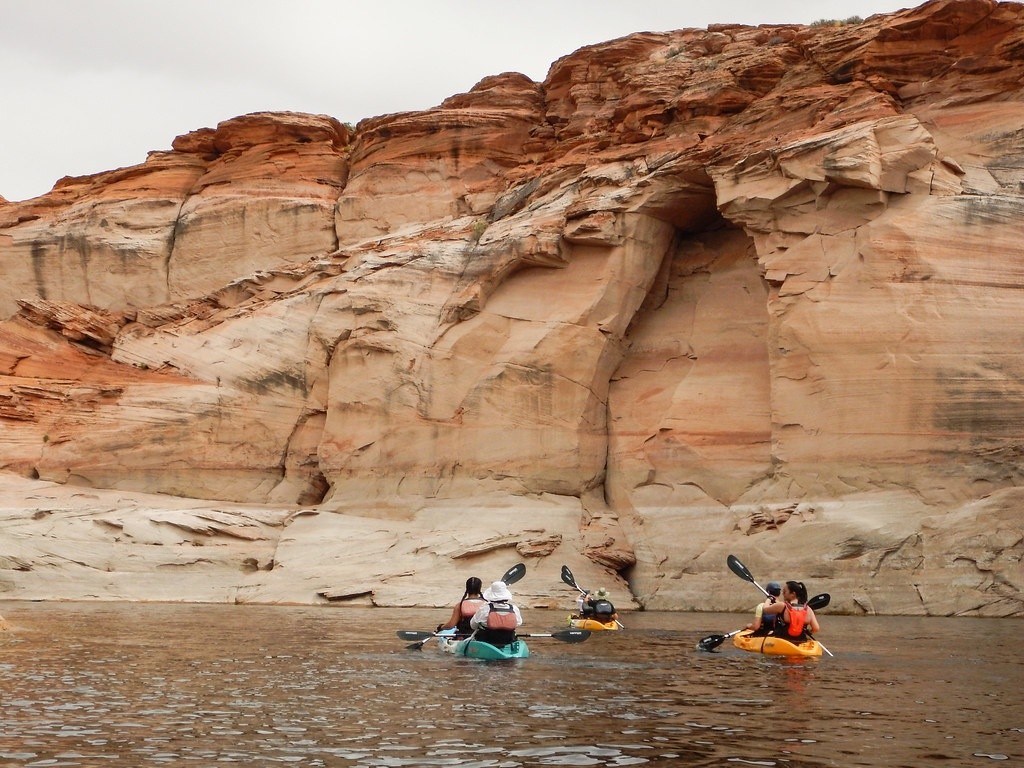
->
<box><xmin>765</xmin><ymin>582</ymin><xmax>781</xmax><ymax>590</ymax></box>
<box><xmin>483</xmin><ymin>582</ymin><xmax>511</xmax><ymax>601</ymax></box>
<box><xmin>594</xmin><ymin>587</ymin><xmax>609</xmax><ymax>600</ymax></box>
<box><xmin>580</xmin><ymin>590</ymin><xmax>593</xmax><ymax>597</ymax></box>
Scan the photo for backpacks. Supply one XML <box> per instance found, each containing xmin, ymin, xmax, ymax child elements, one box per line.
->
<box><xmin>786</xmin><ymin>601</ymin><xmax>808</xmax><ymax>637</ymax></box>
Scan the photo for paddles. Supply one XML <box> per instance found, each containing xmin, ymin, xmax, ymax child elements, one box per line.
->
<box><xmin>727</xmin><ymin>555</ymin><xmax>834</xmax><ymax>658</ymax></box>
<box><xmin>516</xmin><ymin>630</ymin><xmax>591</xmax><ymax>643</ymax></box>
<box><xmin>560</xmin><ymin>572</ymin><xmax>625</xmax><ymax>630</ymax></box>
<box><xmin>562</xmin><ymin>564</ymin><xmax>587</xmax><ymax>597</ymax></box>
<box><xmin>404</xmin><ymin>628</ymin><xmax>442</xmax><ymax>651</ymax></box>
<box><xmin>807</xmin><ymin>593</ymin><xmax>831</xmax><ymax>611</ymax></box>
<box><xmin>699</xmin><ymin>625</ymin><xmax>750</xmax><ymax>650</ymax></box>
<box><xmin>501</xmin><ymin>563</ymin><xmax>526</xmax><ymax>586</ymax></box>
<box><xmin>396</xmin><ymin>630</ymin><xmax>473</xmax><ymax>641</ymax></box>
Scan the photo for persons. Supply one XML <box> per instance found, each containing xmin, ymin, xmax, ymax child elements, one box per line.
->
<box><xmin>576</xmin><ymin>590</ymin><xmax>593</xmax><ymax>618</ymax></box>
<box><xmin>436</xmin><ymin>577</ymin><xmax>487</xmax><ymax>640</ymax></box>
<box><xmin>470</xmin><ymin>582</ymin><xmax>522</xmax><ymax>647</ymax></box>
<box><xmin>762</xmin><ymin>581</ymin><xmax>819</xmax><ymax>647</ymax></box>
<box><xmin>588</xmin><ymin>588</ymin><xmax>619</xmax><ymax>625</ymax></box>
<box><xmin>741</xmin><ymin>582</ymin><xmax>784</xmax><ymax>637</ymax></box>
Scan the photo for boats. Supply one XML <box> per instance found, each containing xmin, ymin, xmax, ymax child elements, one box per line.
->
<box><xmin>566</xmin><ymin>614</ymin><xmax>618</xmax><ymax>631</ymax></box>
<box><xmin>438</xmin><ymin>626</ymin><xmax>530</xmax><ymax>660</ymax></box>
<box><xmin>734</xmin><ymin>628</ymin><xmax>822</xmax><ymax>656</ymax></box>
